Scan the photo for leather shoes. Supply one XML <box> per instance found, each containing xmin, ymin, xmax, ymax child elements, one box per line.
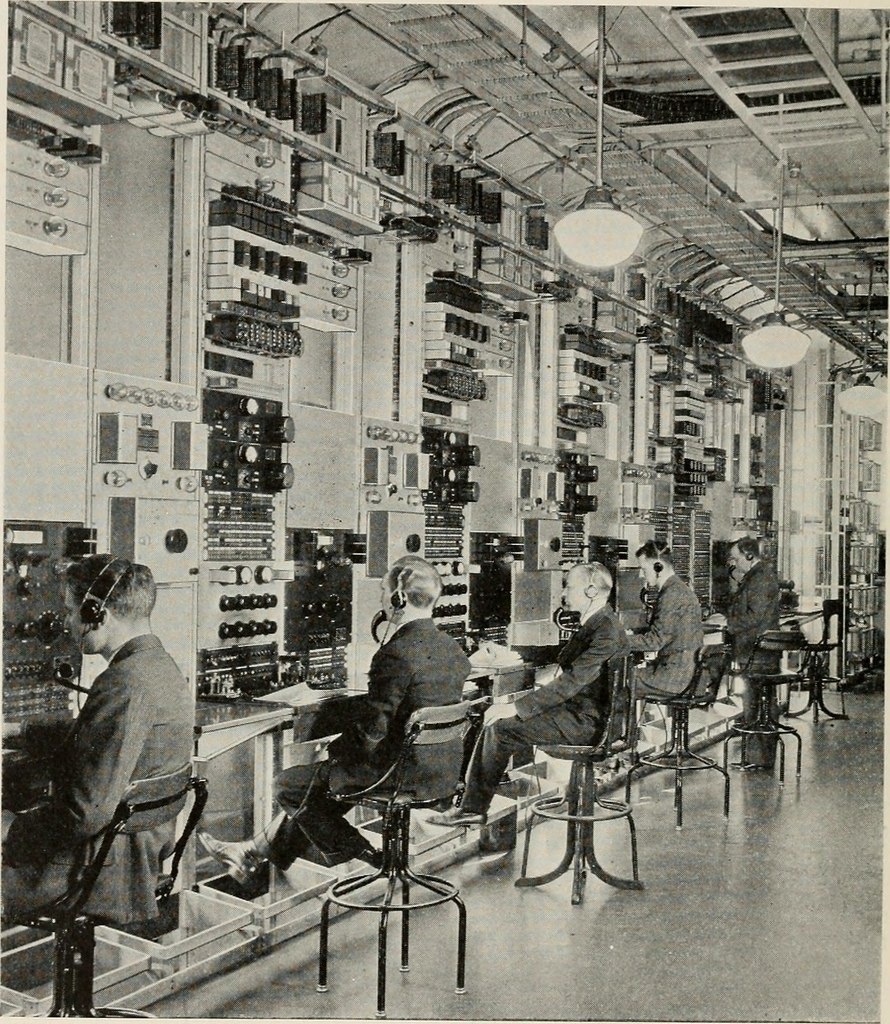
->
<box><xmin>730</xmin><ymin>761</ymin><xmax>771</xmax><ymax>771</ymax></box>
<box><xmin>197</xmin><ymin>833</ymin><xmax>260</xmax><ymax>885</ymax></box>
<box><xmin>425</xmin><ymin>806</ymin><xmax>488</xmax><ymax>825</ymax></box>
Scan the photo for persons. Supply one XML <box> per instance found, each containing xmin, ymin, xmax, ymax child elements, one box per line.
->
<box><xmin>1</xmin><ymin>555</ymin><xmax>194</xmax><ymax>925</ymax></box>
<box><xmin>199</xmin><ymin>556</ymin><xmax>471</xmax><ymax>885</ymax></box>
<box><xmin>424</xmin><ymin>562</ymin><xmax>629</xmax><ymax>828</ymax></box>
<box><xmin>626</xmin><ymin>540</ymin><xmax>703</xmax><ymax>746</ymax></box>
<box><xmin>695</xmin><ymin>537</ymin><xmax>779</xmax><ymax>707</ymax></box>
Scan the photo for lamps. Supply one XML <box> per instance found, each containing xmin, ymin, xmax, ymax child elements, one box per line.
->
<box><xmin>837</xmin><ymin>262</ymin><xmax>887</xmax><ymax>417</ymax></box>
<box><xmin>742</xmin><ymin>94</ymin><xmax>813</xmax><ymax>368</ymax></box>
<box><xmin>551</xmin><ymin>7</ymin><xmax>644</xmax><ymax>267</ymax></box>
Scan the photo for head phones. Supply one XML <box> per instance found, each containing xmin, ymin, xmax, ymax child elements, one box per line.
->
<box><xmin>654</xmin><ymin>541</ymin><xmax>662</xmax><ymax>573</ymax></box>
<box><xmin>586</xmin><ymin>566</ymin><xmax>599</xmax><ymax>600</ymax></box>
<box><xmin>391</xmin><ymin>563</ymin><xmax>418</xmax><ymax>609</ymax></box>
<box><xmin>78</xmin><ymin>557</ymin><xmax>132</xmax><ymax>624</ymax></box>
<box><xmin>739</xmin><ymin>541</ymin><xmax>753</xmax><ymax>561</ymax></box>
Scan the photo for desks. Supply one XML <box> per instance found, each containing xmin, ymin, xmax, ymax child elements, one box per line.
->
<box><xmin>0</xmin><ymin>607</ymin><xmax>823</xmax><ymax>1017</ymax></box>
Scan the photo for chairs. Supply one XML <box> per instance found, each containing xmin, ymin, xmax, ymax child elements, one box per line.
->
<box><xmin>318</xmin><ymin>691</ymin><xmax>500</xmax><ymax>1018</ymax></box>
<box><xmin>723</xmin><ymin>630</ymin><xmax>814</xmax><ymax>785</ymax></box>
<box><xmin>514</xmin><ymin>653</ymin><xmax>647</xmax><ymax>906</ymax></box>
<box><xmin>0</xmin><ymin>775</ymin><xmax>211</xmax><ymax>1024</ymax></box>
<box><xmin>784</xmin><ymin>597</ymin><xmax>854</xmax><ymax>726</ymax></box>
<box><xmin>623</xmin><ymin>641</ymin><xmax>733</xmax><ymax>832</ymax></box>
